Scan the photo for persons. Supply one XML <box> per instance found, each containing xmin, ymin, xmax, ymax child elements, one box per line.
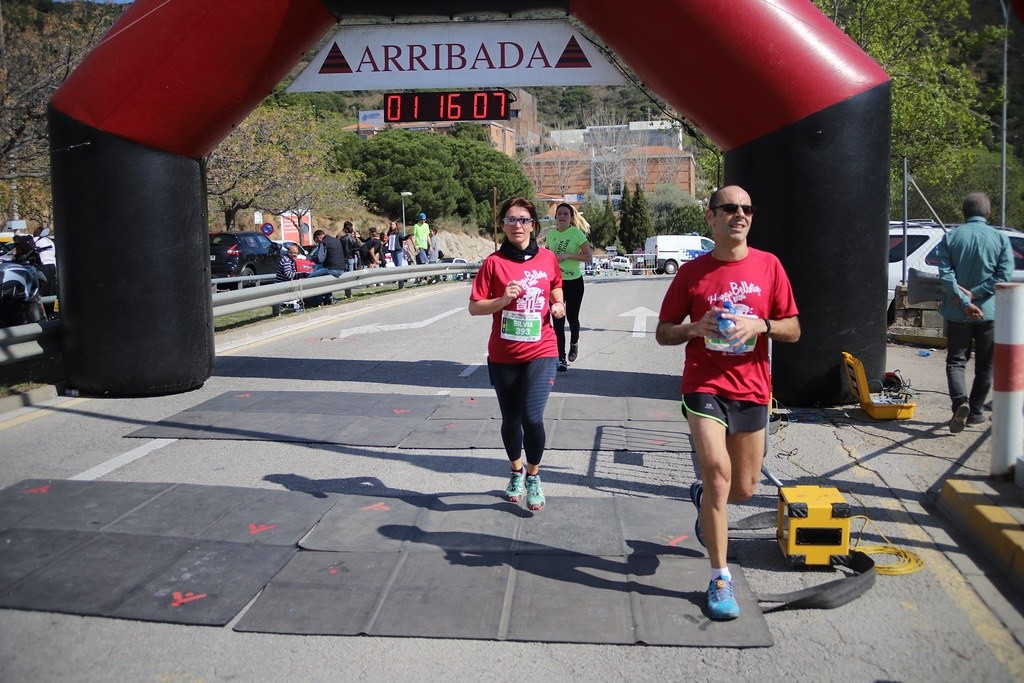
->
<box><xmin>468</xmin><ymin>198</ymin><xmax>566</xmax><ymax>510</ymax></box>
<box><xmin>546</xmin><ymin>203</ymin><xmax>593</xmax><ymax>372</ymax></box>
<box><xmin>275</xmin><ymin>245</ymin><xmax>309</xmax><ymax>282</ymax></box>
<box><xmin>387</xmin><ymin>220</ymin><xmax>413</xmax><ymax>266</ymax></box>
<box><xmin>426</xmin><ymin>226</ymin><xmax>439</xmax><ymax>263</ymax></box>
<box><xmin>32</xmin><ymin>226</ymin><xmax>57</xmax><ymax>317</ymax></box>
<box><xmin>655</xmin><ymin>186</ymin><xmax>801</xmax><ymax>619</ymax></box>
<box><xmin>308</xmin><ymin>230</ymin><xmax>346</xmax><ymax>306</ymax></box>
<box><xmin>411</xmin><ymin>213</ymin><xmax>432</xmax><ymax>282</ymax></box>
<box><xmin>337</xmin><ymin>221</ymin><xmax>389</xmax><ymax>271</ymax></box>
<box><xmin>937</xmin><ymin>193</ymin><xmax>1015</xmax><ymax>434</ymax></box>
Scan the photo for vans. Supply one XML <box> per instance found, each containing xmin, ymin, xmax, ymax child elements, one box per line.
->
<box><xmin>644</xmin><ymin>232</ymin><xmax>716</xmax><ymax>275</ymax></box>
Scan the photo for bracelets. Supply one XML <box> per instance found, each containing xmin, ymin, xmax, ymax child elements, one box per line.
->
<box><xmin>762</xmin><ymin>318</ymin><xmax>771</xmax><ymax>336</ymax></box>
<box><xmin>555</xmin><ymin>301</ymin><xmax>564</xmax><ymax>306</ymax></box>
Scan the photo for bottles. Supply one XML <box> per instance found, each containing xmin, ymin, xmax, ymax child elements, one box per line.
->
<box><xmin>716</xmin><ymin>313</ymin><xmax>747</xmax><ymax>355</ymax></box>
<box><xmin>723</xmin><ymin>301</ymin><xmax>736</xmax><ymax>314</ymax></box>
<box><xmin>918</xmin><ymin>349</ymin><xmax>931</xmax><ymax>357</ymax></box>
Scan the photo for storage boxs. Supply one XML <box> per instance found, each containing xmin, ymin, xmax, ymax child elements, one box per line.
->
<box><xmin>842</xmin><ymin>352</ymin><xmax>916</xmax><ymax>418</ymax></box>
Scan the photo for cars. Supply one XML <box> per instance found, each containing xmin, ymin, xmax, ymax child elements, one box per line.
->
<box><xmin>585</xmin><ymin>257</ymin><xmax>632</xmax><ymax>275</ymax></box>
<box><xmin>272</xmin><ymin>241</ymin><xmax>309</xmax><ymax>261</ymax></box>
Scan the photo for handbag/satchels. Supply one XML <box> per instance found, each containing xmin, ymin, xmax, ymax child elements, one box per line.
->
<box><xmin>438</xmin><ymin>250</ymin><xmax>444</xmax><ymax>259</ymax></box>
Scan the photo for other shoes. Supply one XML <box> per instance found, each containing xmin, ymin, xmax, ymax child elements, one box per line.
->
<box><xmin>949</xmin><ymin>402</ymin><xmax>971</xmax><ymax>434</ymax></box>
<box><xmin>322</xmin><ymin>298</ymin><xmax>333</xmax><ymax>305</ymax></box>
<box><xmin>966</xmin><ymin>411</ymin><xmax>986</xmax><ymax>426</ymax></box>
<box><xmin>308</xmin><ymin>301</ymin><xmax>325</xmax><ymax>307</ymax></box>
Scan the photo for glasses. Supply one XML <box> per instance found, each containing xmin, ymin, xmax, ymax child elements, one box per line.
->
<box><xmin>501</xmin><ymin>216</ymin><xmax>534</xmax><ymax>226</ymax></box>
<box><xmin>421</xmin><ymin>218</ymin><xmax>426</xmax><ymax>220</ymax></box>
<box><xmin>712</xmin><ymin>203</ymin><xmax>756</xmax><ymax>215</ymax></box>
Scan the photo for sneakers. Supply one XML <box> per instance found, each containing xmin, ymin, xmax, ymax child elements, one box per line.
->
<box><xmin>525</xmin><ymin>472</ymin><xmax>546</xmax><ymax>510</ymax></box>
<box><xmin>691</xmin><ymin>480</ymin><xmax>707</xmax><ymax>548</ymax></box>
<box><xmin>505</xmin><ymin>463</ymin><xmax>527</xmax><ymax>502</ymax></box>
<box><xmin>568</xmin><ymin>342</ymin><xmax>578</xmax><ymax>362</ymax></box>
<box><xmin>705</xmin><ymin>574</ymin><xmax>740</xmax><ymax>619</ymax></box>
<box><xmin>557</xmin><ymin>357</ymin><xmax>568</xmax><ymax>371</ymax></box>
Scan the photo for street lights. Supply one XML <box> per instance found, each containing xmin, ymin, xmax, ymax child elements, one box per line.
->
<box><xmin>401</xmin><ymin>191</ymin><xmax>412</xmax><ymax>236</ymax></box>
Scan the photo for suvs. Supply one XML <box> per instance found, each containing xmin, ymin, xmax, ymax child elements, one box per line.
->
<box><xmin>887</xmin><ymin>223</ymin><xmax>1024</xmax><ymax>328</ymax></box>
<box><xmin>208</xmin><ymin>231</ymin><xmax>282</xmax><ymax>288</ymax></box>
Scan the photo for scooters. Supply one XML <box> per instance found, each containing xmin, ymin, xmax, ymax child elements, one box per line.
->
<box><xmin>0</xmin><ymin>227</ymin><xmax>53</xmax><ymax>329</ymax></box>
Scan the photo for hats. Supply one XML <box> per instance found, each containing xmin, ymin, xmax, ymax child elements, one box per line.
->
<box><xmin>418</xmin><ymin>213</ymin><xmax>426</xmax><ymax>219</ymax></box>
